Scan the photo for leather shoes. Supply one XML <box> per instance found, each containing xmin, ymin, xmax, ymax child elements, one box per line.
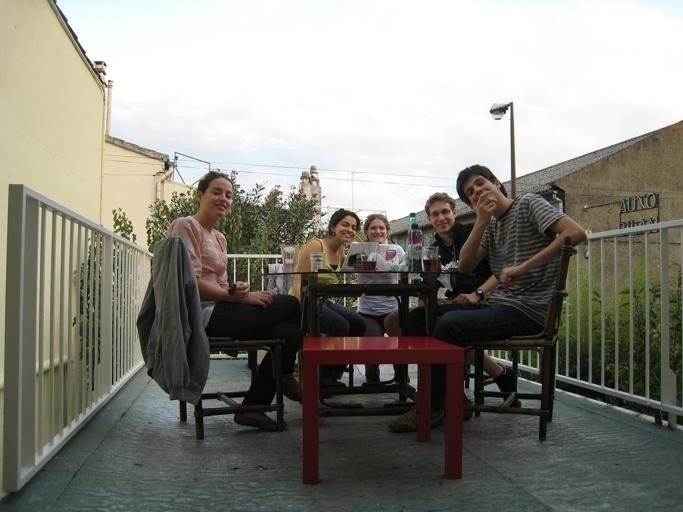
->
<box><xmin>283</xmin><ymin>378</ymin><xmax>301</xmax><ymax>401</ymax></box>
<box><xmin>234</xmin><ymin>412</ymin><xmax>286</xmax><ymax>431</ymax></box>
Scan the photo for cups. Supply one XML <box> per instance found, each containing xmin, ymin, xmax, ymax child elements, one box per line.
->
<box><xmin>266</xmin><ymin>263</ymin><xmax>281</xmax><ymax>274</ymax></box>
<box><xmin>357</xmin><ymin>241</ymin><xmax>378</xmax><ymax>272</ymax></box>
<box><xmin>309</xmin><ymin>252</ymin><xmax>323</xmax><ymax>272</ymax></box>
<box><xmin>281</xmin><ymin>243</ymin><xmax>299</xmax><ymax>273</ymax></box>
<box><xmin>420</xmin><ymin>246</ymin><xmax>438</xmax><ymax>272</ymax></box>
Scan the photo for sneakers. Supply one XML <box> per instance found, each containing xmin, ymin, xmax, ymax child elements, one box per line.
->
<box><xmin>496</xmin><ymin>367</ymin><xmax>517</xmax><ymax>408</ymax></box>
<box><xmin>390</xmin><ymin>407</ymin><xmax>445</xmax><ymax>433</ymax></box>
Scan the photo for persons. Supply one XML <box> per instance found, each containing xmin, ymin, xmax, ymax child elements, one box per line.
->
<box><xmin>289</xmin><ymin>209</ymin><xmax>367</xmax><ymax>386</ymax></box>
<box><xmin>357</xmin><ymin>215</ymin><xmax>410</xmax><ymax>384</ymax></box>
<box><xmin>169</xmin><ymin>171</ymin><xmax>302</xmax><ymax>431</ymax></box>
<box><xmin>388</xmin><ymin>165</ymin><xmax>586</xmax><ymax>433</ymax></box>
<box><xmin>384</xmin><ymin>193</ymin><xmax>521</xmax><ymax>407</ymax></box>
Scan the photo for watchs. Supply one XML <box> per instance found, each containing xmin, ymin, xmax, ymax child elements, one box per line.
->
<box><xmin>475</xmin><ymin>289</ymin><xmax>484</xmax><ymax>297</ymax></box>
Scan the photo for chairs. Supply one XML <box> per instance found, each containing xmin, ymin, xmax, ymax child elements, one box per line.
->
<box><xmin>467</xmin><ymin>236</ymin><xmax>578</xmax><ymax>440</ymax></box>
<box><xmin>293</xmin><ymin>273</ymin><xmax>354</xmax><ymax>389</ymax></box>
<box><xmin>136</xmin><ymin>236</ymin><xmax>287</xmax><ymax>439</ymax></box>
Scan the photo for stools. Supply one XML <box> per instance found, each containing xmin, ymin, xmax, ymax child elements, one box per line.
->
<box><xmin>302</xmin><ymin>337</ymin><xmax>465</xmax><ymax>484</ymax></box>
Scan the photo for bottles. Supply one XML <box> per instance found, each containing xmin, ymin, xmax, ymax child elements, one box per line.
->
<box><xmin>405</xmin><ymin>213</ymin><xmax>415</xmax><ymax>228</ymax></box>
<box><xmin>407</xmin><ymin>223</ymin><xmax>422</xmax><ymax>271</ymax></box>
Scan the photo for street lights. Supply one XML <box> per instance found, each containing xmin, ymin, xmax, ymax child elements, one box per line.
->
<box><xmin>489</xmin><ymin>101</ymin><xmax>517</xmax><ymax>200</ymax></box>
